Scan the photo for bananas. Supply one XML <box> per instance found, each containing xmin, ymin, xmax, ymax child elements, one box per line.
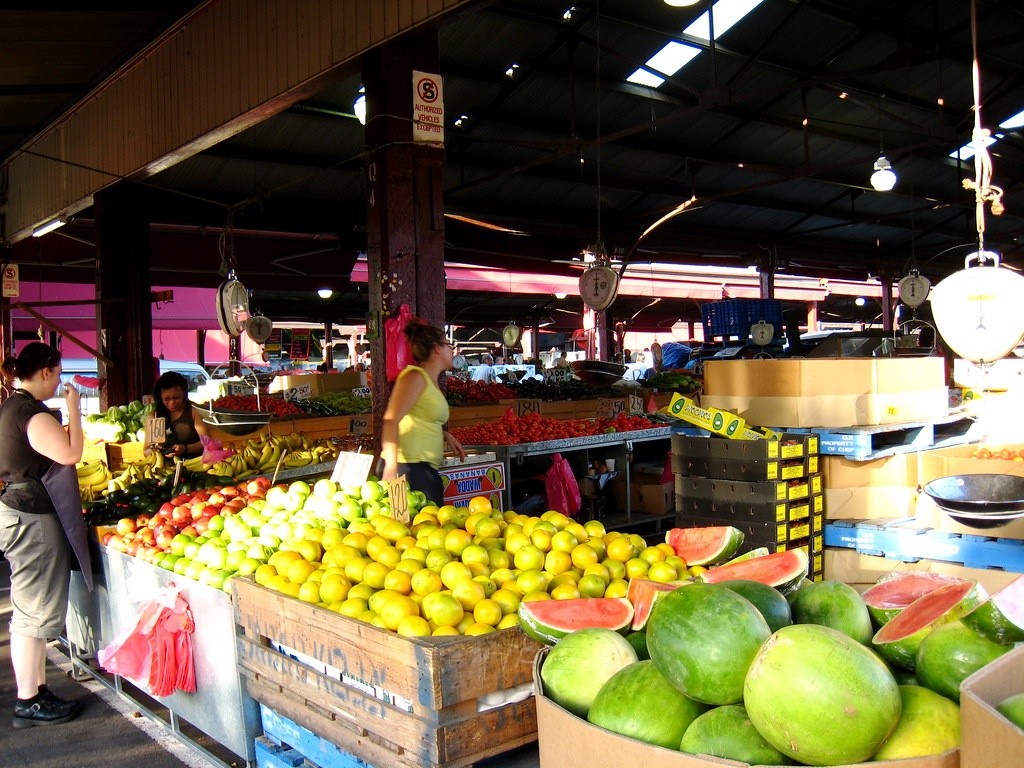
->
<box><xmin>184</xmin><ymin>431</ymin><xmax>340</xmax><ymax>480</ymax></box>
<box><xmin>77</xmin><ymin>447</ymin><xmax>181</xmax><ymax>502</ymax></box>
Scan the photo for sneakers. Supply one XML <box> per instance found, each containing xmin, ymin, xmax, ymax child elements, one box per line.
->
<box><xmin>12</xmin><ymin>697</ymin><xmax>77</xmax><ymax>729</ymax></box>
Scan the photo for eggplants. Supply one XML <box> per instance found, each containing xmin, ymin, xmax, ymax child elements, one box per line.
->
<box><xmin>505</xmin><ymin>377</ymin><xmax>625</xmax><ymax>403</ymax></box>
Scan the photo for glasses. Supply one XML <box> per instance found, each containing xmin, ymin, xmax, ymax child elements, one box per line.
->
<box><xmin>440</xmin><ymin>341</ymin><xmax>454</xmax><ymax>349</ymax></box>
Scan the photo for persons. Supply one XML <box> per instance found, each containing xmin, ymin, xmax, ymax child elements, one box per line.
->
<box><xmin>152</xmin><ymin>372</ymin><xmax>210</xmax><ymax>462</ymax></box>
<box><xmin>472</xmin><ymin>347</ymin><xmax>649</xmax><ymax>384</ymax></box>
<box><xmin>375</xmin><ymin>318</ymin><xmax>464</xmax><ymax>509</ymax></box>
<box><xmin>0</xmin><ymin>342</ymin><xmax>94</xmax><ymax>728</ymax></box>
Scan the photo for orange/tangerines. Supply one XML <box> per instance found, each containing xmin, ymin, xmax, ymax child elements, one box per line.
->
<box><xmin>255</xmin><ymin>496</ymin><xmax>687</xmax><ymax>638</ymax></box>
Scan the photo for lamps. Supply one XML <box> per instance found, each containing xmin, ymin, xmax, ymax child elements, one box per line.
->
<box><xmin>870</xmin><ymin>94</ymin><xmax>896</xmax><ymax>192</ymax></box>
<box><xmin>32</xmin><ymin>214</ymin><xmax>67</xmax><ymax>237</ymax></box>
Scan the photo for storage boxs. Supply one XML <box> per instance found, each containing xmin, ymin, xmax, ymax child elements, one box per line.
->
<box><xmin>206</xmin><ymin>372</ymin><xmax>702</xmax><ymax>452</ymax></box>
<box><xmin>704</xmin><ymin>357</ymin><xmax>948</xmax><ymax>427</ymax></box>
<box><xmin>233</xmin><ymin>576</ymin><xmax>1024</xmax><ymax>768</ymax></box>
<box><xmin>702</xmin><ymin>297</ymin><xmax>782</xmax><ymax>342</ymax></box>
<box><xmin>612</xmin><ymin>475</ymin><xmax>674</xmax><ymax>515</ymax></box>
<box><xmin>74</xmin><ymin>440</ymin><xmax>144</xmax><ymax>471</ymax></box>
<box><xmin>668</xmin><ymin>391</ymin><xmax>825</xmax><ymax>583</ymax></box>
<box><xmin>438</xmin><ymin>461</ymin><xmax>505</xmax><ymax>512</ymax></box>
<box><xmin>819</xmin><ymin>445</ymin><xmax>1024</xmax><ymax>597</ymax></box>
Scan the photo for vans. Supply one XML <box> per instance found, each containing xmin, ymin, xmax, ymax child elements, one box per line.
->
<box><xmin>54</xmin><ymin>357</ymin><xmax>213</xmax><ymax>398</ymax></box>
<box><xmin>187</xmin><ymin>361</ymin><xmax>273</xmax><ymax>380</ymax></box>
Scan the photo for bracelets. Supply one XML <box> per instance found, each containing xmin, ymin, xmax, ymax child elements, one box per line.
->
<box><xmin>184</xmin><ymin>444</ymin><xmax>188</xmax><ymax>454</ymax></box>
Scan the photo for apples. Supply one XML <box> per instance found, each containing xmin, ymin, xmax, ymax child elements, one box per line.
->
<box><xmin>102</xmin><ymin>475</ymin><xmax>436</xmax><ymax>588</ymax></box>
<box><xmin>308</xmin><ymin>392</ymin><xmax>374</xmax><ymax>451</ymax></box>
<box><xmin>204</xmin><ymin>395</ymin><xmax>305</xmax><ymax>417</ymax></box>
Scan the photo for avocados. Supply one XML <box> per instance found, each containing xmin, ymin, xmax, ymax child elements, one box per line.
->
<box><xmin>81</xmin><ymin>470</ymin><xmax>235</xmax><ymax>526</ymax></box>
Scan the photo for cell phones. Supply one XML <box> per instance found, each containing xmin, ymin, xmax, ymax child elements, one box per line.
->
<box><xmin>164</xmin><ymin>448</ymin><xmax>175</xmax><ymax>455</ymax></box>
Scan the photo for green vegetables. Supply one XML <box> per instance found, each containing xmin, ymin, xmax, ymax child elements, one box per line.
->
<box><xmin>639</xmin><ymin>371</ymin><xmax>703</xmax><ymax>397</ymax></box>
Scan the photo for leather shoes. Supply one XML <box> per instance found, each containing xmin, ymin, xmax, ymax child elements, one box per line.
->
<box><xmin>38</xmin><ymin>689</ymin><xmax>84</xmax><ymax>713</ymax></box>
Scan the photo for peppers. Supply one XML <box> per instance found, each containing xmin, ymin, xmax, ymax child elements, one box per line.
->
<box><xmin>442</xmin><ymin>376</ymin><xmax>517</xmax><ymax>404</ymax></box>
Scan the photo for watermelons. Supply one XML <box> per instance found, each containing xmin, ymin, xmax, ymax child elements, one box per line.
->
<box><xmin>665</xmin><ymin>526</ymin><xmax>745</xmax><ymax>565</ymax></box>
<box><xmin>519</xmin><ymin>548</ymin><xmax>1024</xmax><ymax>766</ymax></box>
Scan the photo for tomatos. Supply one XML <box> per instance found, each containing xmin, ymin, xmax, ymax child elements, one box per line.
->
<box><xmin>447</xmin><ymin>407</ymin><xmax>668</xmax><ymax>446</ymax></box>
